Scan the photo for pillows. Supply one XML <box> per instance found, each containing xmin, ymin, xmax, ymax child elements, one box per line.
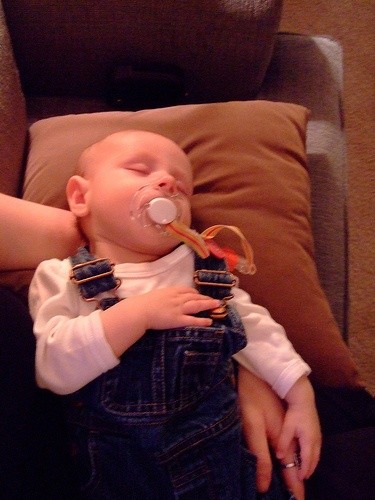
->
<box><xmin>11</xmin><ymin>99</ymin><xmax>368</xmax><ymax>391</ymax></box>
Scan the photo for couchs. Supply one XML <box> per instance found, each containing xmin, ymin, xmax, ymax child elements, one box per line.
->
<box><xmin>0</xmin><ymin>0</ymin><xmax>372</xmax><ymax>389</ymax></box>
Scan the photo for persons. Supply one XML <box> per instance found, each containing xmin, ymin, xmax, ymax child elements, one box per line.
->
<box><xmin>28</xmin><ymin>128</ymin><xmax>323</xmax><ymax>500</ymax></box>
<box><xmin>0</xmin><ymin>192</ymin><xmax>308</xmax><ymax>500</ymax></box>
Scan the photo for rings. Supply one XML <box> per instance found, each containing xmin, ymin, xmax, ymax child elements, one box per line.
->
<box><xmin>283</xmin><ymin>460</ymin><xmax>299</xmax><ymax>469</ymax></box>
